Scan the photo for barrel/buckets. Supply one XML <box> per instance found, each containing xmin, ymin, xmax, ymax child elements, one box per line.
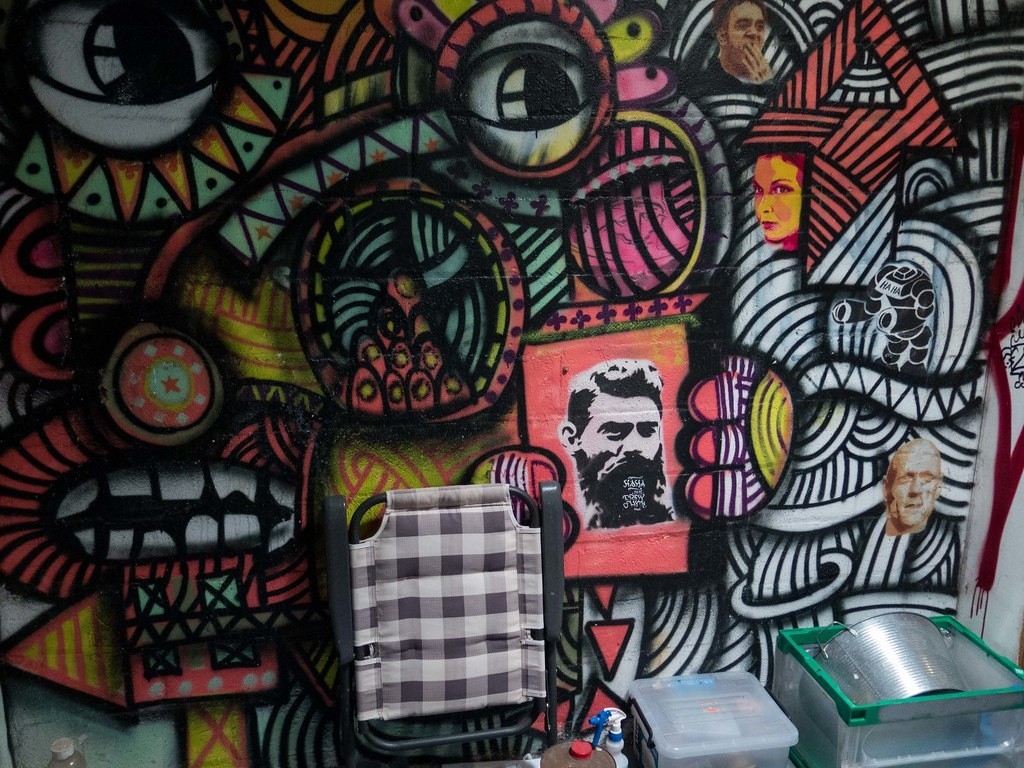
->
<box><xmin>800</xmin><ymin>611</ymin><xmax>970</xmax><ymax>757</ymax></box>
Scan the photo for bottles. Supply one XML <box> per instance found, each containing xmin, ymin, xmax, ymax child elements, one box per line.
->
<box><xmin>47</xmin><ymin>737</ymin><xmax>87</xmax><ymax>768</ymax></box>
<box><xmin>540</xmin><ymin>740</ymin><xmax>617</xmax><ymax>768</ymax></box>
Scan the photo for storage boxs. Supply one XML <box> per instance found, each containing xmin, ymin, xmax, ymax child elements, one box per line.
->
<box><xmin>628</xmin><ymin>671</ymin><xmax>799</xmax><ymax>768</ymax></box>
<box><xmin>769</xmin><ymin>614</ymin><xmax>1024</xmax><ymax>768</ymax></box>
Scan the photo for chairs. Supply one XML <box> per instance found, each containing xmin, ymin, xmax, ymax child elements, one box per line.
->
<box><xmin>321</xmin><ymin>478</ymin><xmax>563</xmax><ymax>768</ymax></box>
